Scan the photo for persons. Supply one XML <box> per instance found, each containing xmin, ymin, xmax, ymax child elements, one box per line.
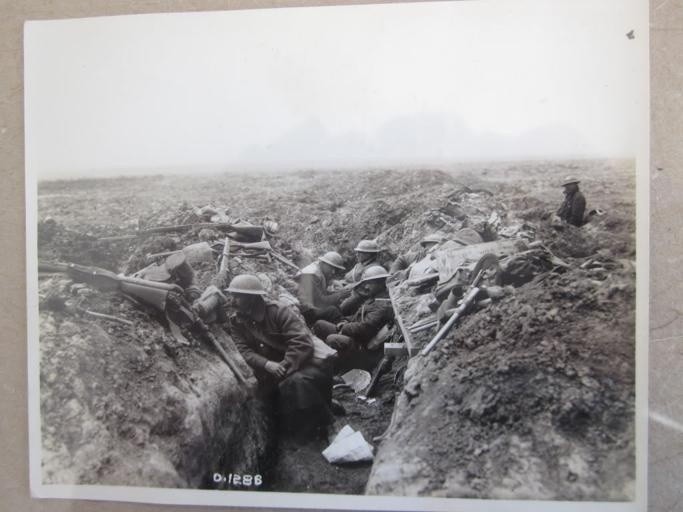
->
<box><xmin>557</xmin><ymin>176</ymin><xmax>585</xmax><ymax>228</ymax></box>
<box><xmin>295</xmin><ymin>228</ymin><xmax>390</xmax><ymax>375</ymax></box>
<box><xmin>226</xmin><ymin>275</ymin><xmax>338</xmax><ymax>439</ymax></box>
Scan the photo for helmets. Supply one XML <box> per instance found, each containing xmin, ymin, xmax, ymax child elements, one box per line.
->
<box><xmin>561</xmin><ymin>176</ymin><xmax>580</xmax><ymax>186</ymax></box>
<box><xmin>420</xmin><ymin>234</ymin><xmax>440</xmax><ymax>247</ymax></box>
<box><xmin>355</xmin><ymin>240</ymin><xmax>381</xmax><ymax>253</ymax></box>
<box><xmin>361</xmin><ymin>266</ymin><xmax>389</xmax><ymax>282</ymax></box>
<box><xmin>223</xmin><ymin>275</ymin><xmax>265</xmax><ymax>295</ymax></box>
<box><xmin>318</xmin><ymin>252</ymin><xmax>346</xmax><ymax>271</ymax></box>
